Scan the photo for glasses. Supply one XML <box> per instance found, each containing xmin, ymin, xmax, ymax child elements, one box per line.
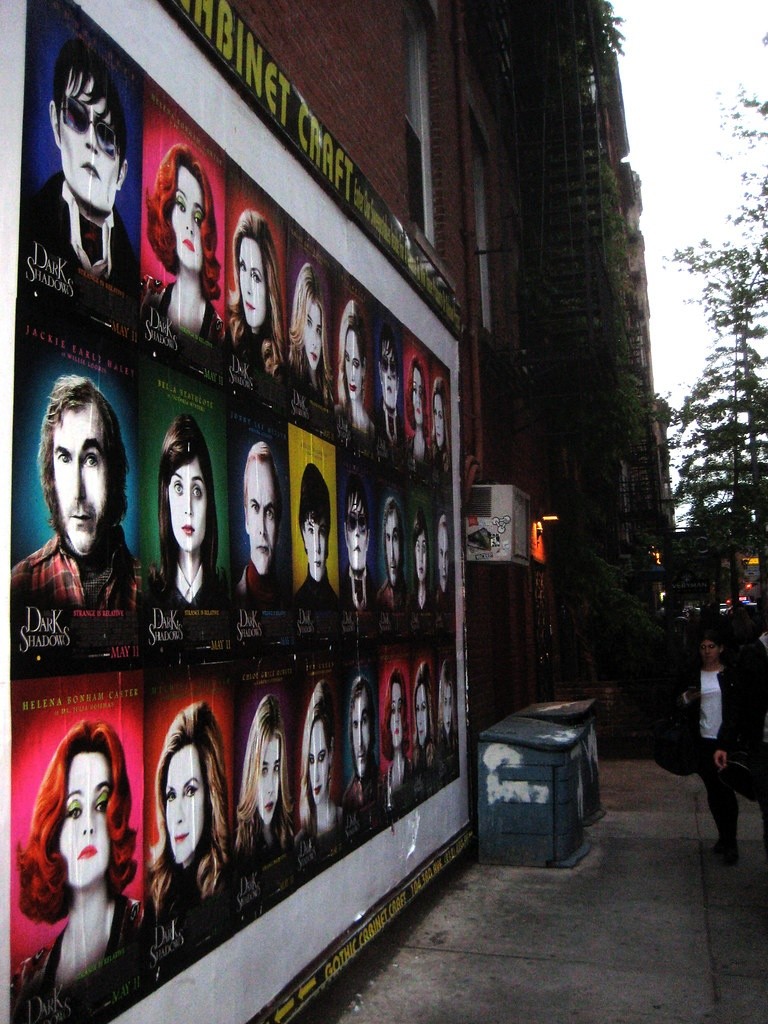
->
<box><xmin>65</xmin><ymin>96</ymin><xmax>119</xmax><ymax>156</ymax></box>
<box><xmin>348</xmin><ymin>512</ymin><xmax>367</xmax><ymax>531</ymax></box>
<box><xmin>699</xmin><ymin>644</ymin><xmax>719</xmax><ymax>650</ymax></box>
<box><xmin>379</xmin><ymin>355</ymin><xmax>398</xmax><ymax>374</ymax></box>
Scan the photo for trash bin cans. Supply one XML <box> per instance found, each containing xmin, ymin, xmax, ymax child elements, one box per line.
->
<box><xmin>477</xmin><ymin>699</ymin><xmax>607</xmax><ymax>868</ymax></box>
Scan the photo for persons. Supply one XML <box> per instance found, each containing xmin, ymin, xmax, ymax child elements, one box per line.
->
<box><xmin>35</xmin><ymin>40</ymin><xmax>133</xmax><ymax>291</ymax></box>
<box><xmin>345</xmin><ymin>675</ymin><xmax>381</xmax><ymax>811</ymax></box>
<box><xmin>699</xmin><ymin>598</ymin><xmax>768</xmax><ymax>654</ymax></box>
<box><xmin>236</xmin><ymin>693</ymin><xmax>296</xmax><ymax>859</ymax></box>
<box><xmin>10</xmin><ymin>373</ymin><xmax>140</xmax><ymax>609</ymax></box>
<box><xmin>674</xmin><ymin>601</ymin><xmax>768</xmax><ymax>866</ymax></box>
<box><xmin>376</xmin><ymin>326</ymin><xmax>405</xmax><ymax>449</ymax></box>
<box><xmin>299</xmin><ymin>680</ymin><xmax>346</xmax><ymax>844</ymax></box>
<box><xmin>147</xmin><ymin>414</ymin><xmax>230</xmax><ymax>608</ymax></box>
<box><xmin>407</xmin><ymin>354</ymin><xmax>430</xmax><ymax>461</ymax></box>
<box><xmin>383</xmin><ymin>659</ymin><xmax>458</xmax><ymax>791</ymax></box>
<box><xmin>151</xmin><ymin>701</ymin><xmax>231</xmax><ymax>918</ymax></box>
<box><xmin>289</xmin><ymin>261</ymin><xmax>332</xmax><ymax>404</ymax></box>
<box><xmin>432</xmin><ymin>376</ymin><xmax>452</xmax><ymax>475</ymax></box>
<box><xmin>379</xmin><ymin>497</ymin><xmax>455</xmax><ymax>611</ymax></box>
<box><xmin>294</xmin><ymin>463</ymin><xmax>339</xmax><ymax>607</ymax></box>
<box><xmin>237</xmin><ymin>441</ymin><xmax>288</xmax><ymax>609</ymax></box>
<box><xmin>11</xmin><ymin>721</ymin><xmax>149</xmax><ymax>1024</ymax></box>
<box><xmin>336</xmin><ymin>300</ymin><xmax>374</xmax><ymax>433</ymax></box>
<box><xmin>228</xmin><ymin>209</ymin><xmax>284</xmax><ymax>383</ymax></box>
<box><xmin>145</xmin><ymin>144</ymin><xmax>226</xmax><ymax>346</ymax></box>
<box><xmin>343</xmin><ymin>477</ymin><xmax>378</xmax><ymax>611</ymax></box>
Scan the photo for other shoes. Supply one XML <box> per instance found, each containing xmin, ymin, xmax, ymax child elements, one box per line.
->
<box><xmin>714</xmin><ymin>839</ymin><xmax>724</xmax><ymax>853</ymax></box>
<box><xmin>723</xmin><ymin>841</ymin><xmax>738</xmax><ymax>864</ymax></box>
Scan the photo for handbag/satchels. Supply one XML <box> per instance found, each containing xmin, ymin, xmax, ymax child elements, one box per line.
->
<box><xmin>655</xmin><ymin>671</ymin><xmax>700</xmax><ymax>776</ymax></box>
<box><xmin>717</xmin><ymin>751</ymin><xmax>757</xmax><ymax>801</ymax></box>
